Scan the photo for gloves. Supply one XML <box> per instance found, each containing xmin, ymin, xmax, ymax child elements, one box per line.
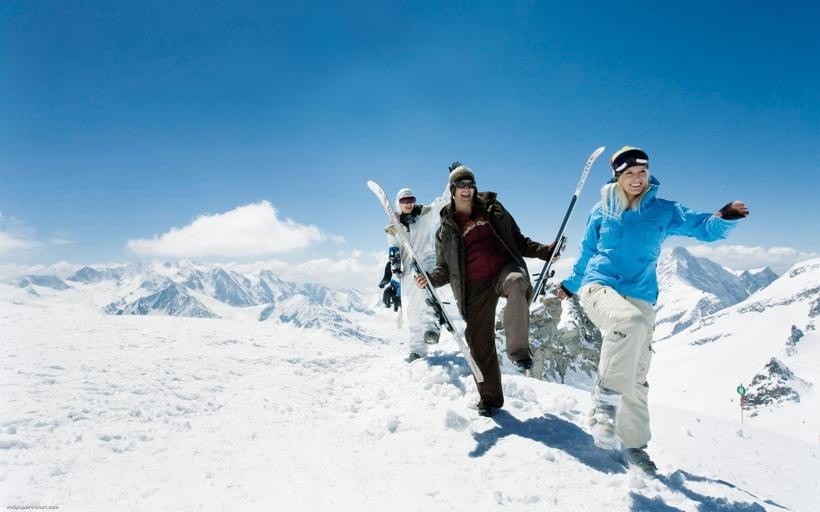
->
<box><xmin>448</xmin><ymin>161</ymin><xmax>461</xmax><ymax>172</ymax></box>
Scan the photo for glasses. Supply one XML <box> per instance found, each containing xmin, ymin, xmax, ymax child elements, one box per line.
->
<box><xmin>399</xmin><ymin>197</ymin><xmax>417</xmax><ymax>204</ymax></box>
<box><xmin>453</xmin><ymin>181</ymin><xmax>476</xmax><ymax>189</ymax></box>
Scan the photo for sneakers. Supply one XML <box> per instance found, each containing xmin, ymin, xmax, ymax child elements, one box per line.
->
<box><xmin>478</xmin><ymin>405</ymin><xmax>495</xmax><ymax>417</ymax></box>
<box><xmin>424</xmin><ymin>331</ymin><xmax>440</xmax><ymax>344</ymax></box>
<box><xmin>589</xmin><ymin>401</ymin><xmax>618</xmax><ymax>450</ymax></box>
<box><xmin>512</xmin><ymin>354</ymin><xmax>533</xmax><ymax>370</ymax></box>
<box><xmin>619</xmin><ymin>446</ymin><xmax>656</xmax><ymax>479</ymax></box>
<box><xmin>409</xmin><ymin>353</ymin><xmax>420</xmax><ymax>362</ymax></box>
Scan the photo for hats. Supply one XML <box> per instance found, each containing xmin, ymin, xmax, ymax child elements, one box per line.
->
<box><xmin>449</xmin><ymin>166</ymin><xmax>475</xmax><ymax>187</ymax></box>
<box><xmin>398</xmin><ymin>188</ymin><xmax>416</xmax><ymax>199</ymax></box>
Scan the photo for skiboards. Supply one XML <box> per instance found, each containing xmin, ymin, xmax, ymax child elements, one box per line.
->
<box><xmin>366</xmin><ymin>146</ymin><xmax>605</xmax><ymax>383</ymax></box>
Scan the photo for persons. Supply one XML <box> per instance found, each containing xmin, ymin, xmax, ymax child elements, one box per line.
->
<box><xmin>382</xmin><ymin>160</ymin><xmax>465</xmax><ymax>364</ymax></box>
<box><xmin>554</xmin><ymin>145</ymin><xmax>751</xmax><ymax>478</ymax></box>
<box><xmin>412</xmin><ymin>165</ymin><xmax>562</xmax><ymax>416</ymax></box>
